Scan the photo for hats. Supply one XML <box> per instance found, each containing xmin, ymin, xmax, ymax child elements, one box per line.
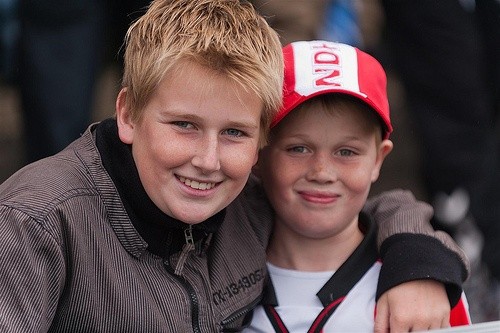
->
<box><xmin>270</xmin><ymin>40</ymin><xmax>393</xmax><ymax>141</ymax></box>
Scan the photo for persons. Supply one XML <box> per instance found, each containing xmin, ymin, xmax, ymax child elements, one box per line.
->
<box><xmin>244</xmin><ymin>0</ymin><xmax>429</xmax><ymax>204</ymax></box>
<box><xmin>0</xmin><ymin>0</ymin><xmax>468</xmax><ymax>332</ymax></box>
<box><xmin>237</xmin><ymin>39</ymin><xmax>475</xmax><ymax>332</ymax></box>
<box><xmin>0</xmin><ymin>1</ymin><xmax>151</xmax><ymax>184</ymax></box>
